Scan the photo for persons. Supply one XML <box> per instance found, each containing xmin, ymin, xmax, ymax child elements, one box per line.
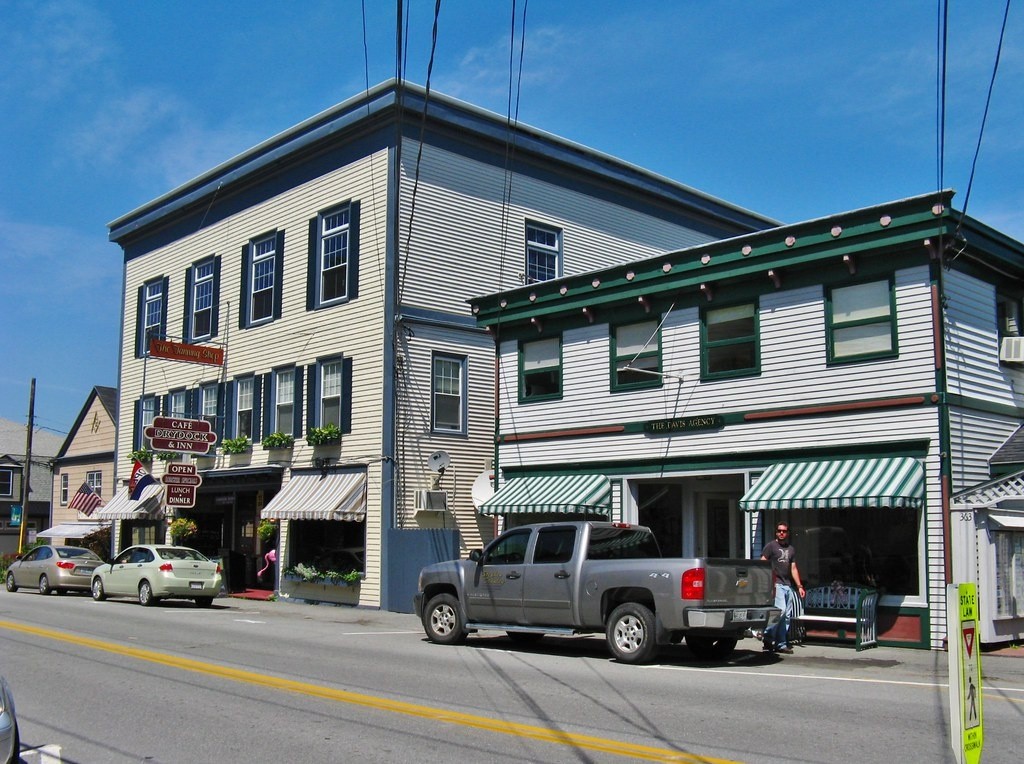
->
<box><xmin>759</xmin><ymin>522</ymin><xmax>808</xmax><ymax>655</ymax></box>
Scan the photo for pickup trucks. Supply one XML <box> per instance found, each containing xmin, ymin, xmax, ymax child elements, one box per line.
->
<box><xmin>412</xmin><ymin>520</ymin><xmax>781</xmax><ymax>665</ymax></box>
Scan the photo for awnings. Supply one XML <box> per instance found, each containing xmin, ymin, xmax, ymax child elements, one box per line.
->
<box><xmin>35</xmin><ymin>522</ymin><xmax>112</xmax><ymax>539</ymax></box>
<box><xmin>98</xmin><ymin>484</ymin><xmax>166</xmax><ymax>520</ymax></box>
<box><xmin>735</xmin><ymin>455</ymin><xmax>924</xmax><ymax>509</ymax></box>
<box><xmin>479</xmin><ymin>474</ymin><xmax>614</xmax><ymax>514</ymax></box>
<box><xmin>260</xmin><ymin>471</ymin><xmax>367</xmax><ymax>522</ymax></box>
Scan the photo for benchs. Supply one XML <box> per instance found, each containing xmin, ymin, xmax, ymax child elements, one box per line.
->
<box><xmin>790</xmin><ymin>580</ymin><xmax>884</xmax><ymax>651</ymax></box>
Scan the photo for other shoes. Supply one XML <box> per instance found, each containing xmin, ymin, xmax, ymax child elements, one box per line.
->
<box><xmin>762</xmin><ymin>642</ymin><xmax>793</xmax><ymax>654</ymax></box>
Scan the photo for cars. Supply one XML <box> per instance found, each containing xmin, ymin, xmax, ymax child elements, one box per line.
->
<box><xmin>88</xmin><ymin>543</ymin><xmax>224</xmax><ymax>609</ymax></box>
<box><xmin>7</xmin><ymin>543</ymin><xmax>108</xmax><ymax>596</ymax></box>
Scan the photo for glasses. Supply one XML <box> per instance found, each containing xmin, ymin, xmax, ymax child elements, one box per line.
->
<box><xmin>776</xmin><ymin>529</ymin><xmax>788</xmax><ymax>533</ymax></box>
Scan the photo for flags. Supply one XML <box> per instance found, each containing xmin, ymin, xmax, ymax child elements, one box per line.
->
<box><xmin>68</xmin><ymin>482</ymin><xmax>100</xmax><ymax>516</ymax></box>
<box><xmin>128</xmin><ymin>459</ymin><xmax>155</xmax><ymax>500</ymax></box>
